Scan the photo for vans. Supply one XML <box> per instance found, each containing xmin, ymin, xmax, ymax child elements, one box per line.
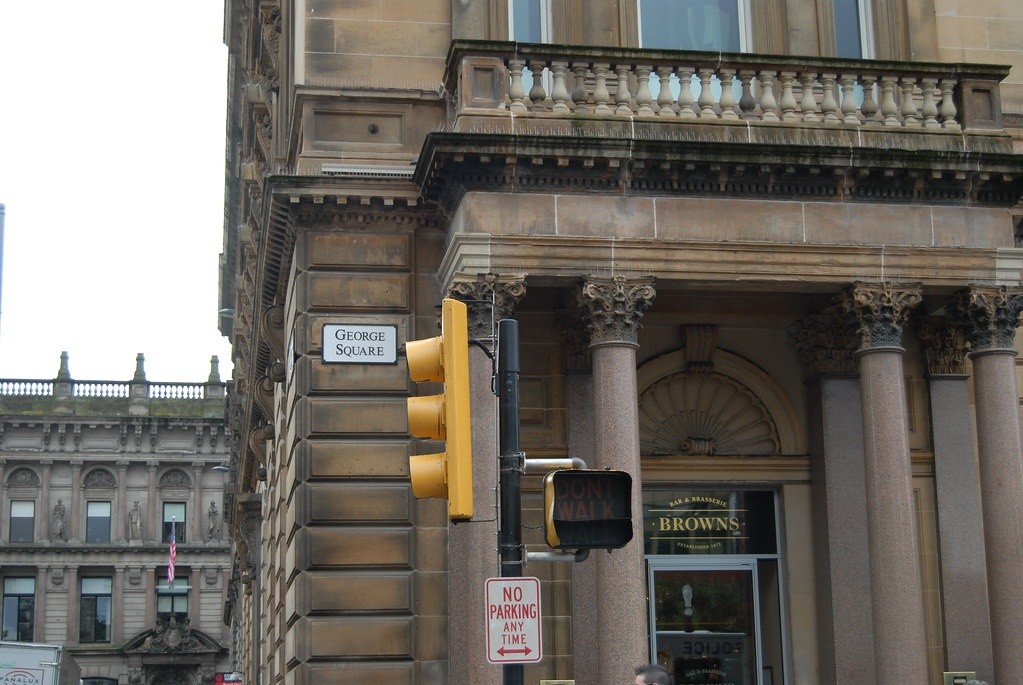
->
<box><xmin>656</xmin><ymin>631</ymin><xmax>754</xmax><ymax>685</ymax></box>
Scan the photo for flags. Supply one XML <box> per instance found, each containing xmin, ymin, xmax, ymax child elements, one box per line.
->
<box><xmin>167</xmin><ymin>519</ymin><xmax>176</xmax><ymax>588</ymax></box>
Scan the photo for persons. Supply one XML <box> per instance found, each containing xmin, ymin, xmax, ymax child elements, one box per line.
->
<box><xmin>179</xmin><ymin>618</ymin><xmax>192</xmax><ymax>644</ymax></box>
<box><xmin>53</xmin><ymin>498</ymin><xmax>67</xmax><ymax>540</ymax></box>
<box><xmin>153</xmin><ymin>619</ymin><xmax>168</xmax><ymax>645</ymax></box>
<box><xmin>207</xmin><ymin>500</ymin><xmax>221</xmax><ymax>542</ymax></box>
<box><xmin>128</xmin><ymin>500</ymin><xmax>144</xmax><ymax>541</ymax></box>
<box><xmin>634</xmin><ymin>664</ymin><xmax>668</xmax><ymax>685</ymax></box>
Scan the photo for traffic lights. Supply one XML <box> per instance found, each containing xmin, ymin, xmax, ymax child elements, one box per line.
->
<box><xmin>543</xmin><ymin>465</ymin><xmax>633</xmax><ymax>551</ymax></box>
<box><xmin>405</xmin><ymin>298</ymin><xmax>474</xmax><ymax>526</ymax></box>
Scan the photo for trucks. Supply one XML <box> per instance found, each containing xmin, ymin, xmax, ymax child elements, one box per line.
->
<box><xmin>0</xmin><ymin>641</ymin><xmax>83</xmax><ymax>685</ymax></box>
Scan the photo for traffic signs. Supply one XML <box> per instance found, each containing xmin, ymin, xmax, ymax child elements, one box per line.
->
<box><xmin>484</xmin><ymin>576</ymin><xmax>543</xmax><ymax>664</ymax></box>
